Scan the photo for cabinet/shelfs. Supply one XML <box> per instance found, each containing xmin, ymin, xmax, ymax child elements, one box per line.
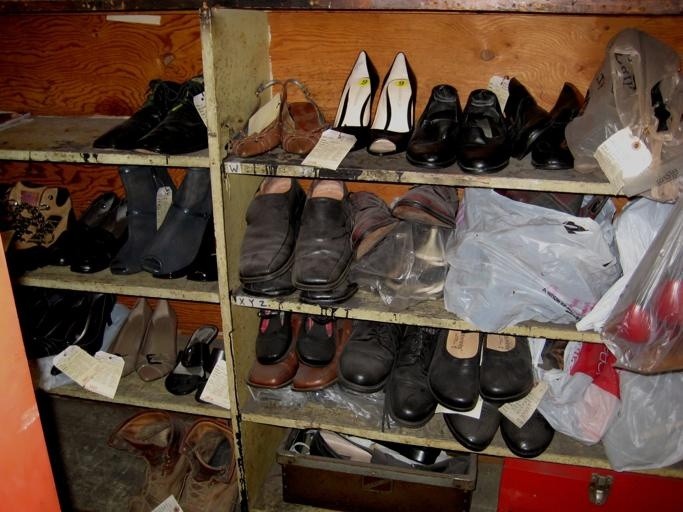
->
<box><xmin>1</xmin><ymin>0</ymin><xmax>682</xmax><ymax>511</ymax></box>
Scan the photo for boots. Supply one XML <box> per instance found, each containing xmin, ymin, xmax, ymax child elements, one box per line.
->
<box><xmin>178</xmin><ymin>418</ymin><xmax>237</xmax><ymax>512</ymax></box>
<box><xmin>106</xmin><ymin>409</ymin><xmax>190</xmax><ymax>512</ymax></box>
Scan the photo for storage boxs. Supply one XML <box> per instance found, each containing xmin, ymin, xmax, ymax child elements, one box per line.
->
<box><xmin>276</xmin><ymin>426</ymin><xmax>479</xmax><ymax>512</ymax></box>
